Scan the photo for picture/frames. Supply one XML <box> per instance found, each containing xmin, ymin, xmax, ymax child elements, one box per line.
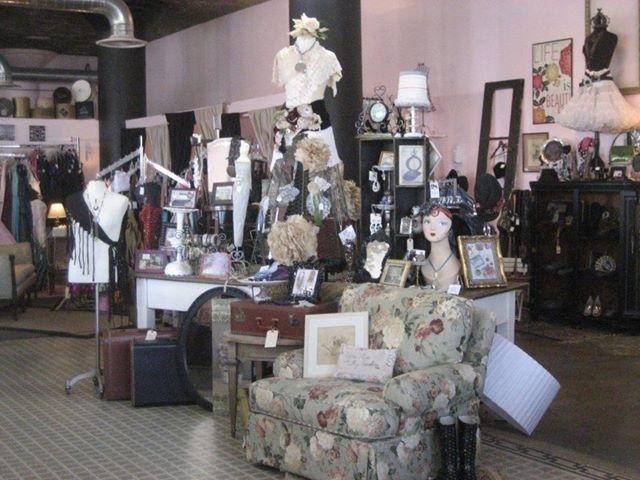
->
<box><xmin>282</xmin><ymin>258</ymin><xmax>324</xmax><ymax>304</ymax></box>
<box><xmin>302</xmin><ymin>312</ymin><xmax>371</xmax><ymax>379</ymax></box>
<box><xmin>132</xmin><ymin>181</ymin><xmax>236</xmax><ymax>280</ymax></box>
<box><xmin>394</xmin><ymin>138</ymin><xmax>429</xmax><ymax>190</ymax></box>
<box><xmin>531</xmin><ymin>36</ymin><xmax>574</xmax><ymax>125</ymax></box>
<box><xmin>522</xmin><ymin>132</ymin><xmax>549</xmax><ymax>173</ymax></box>
<box><xmin>456</xmin><ymin>235</ymin><xmax>508</xmax><ymax>289</ymax></box>
<box><xmin>377</xmin><ymin>258</ymin><xmax>411</xmax><ymax>288</ymax></box>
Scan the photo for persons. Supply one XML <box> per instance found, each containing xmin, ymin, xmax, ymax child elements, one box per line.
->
<box><xmin>554</xmin><ymin>11</ymin><xmax>640</xmax><ymax>135</ymax></box>
<box><xmin>414</xmin><ymin>199</ymin><xmax>462</xmax><ymax>289</ymax></box>
<box><xmin>250</xmin><ymin>13</ymin><xmax>358</xmax><ymax>281</ymax></box>
<box><xmin>64</xmin><ymin>180</ymin><xmax>129</xmax><ymax>283</ymax></box>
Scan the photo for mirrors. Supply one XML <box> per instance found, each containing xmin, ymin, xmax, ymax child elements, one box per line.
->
<box><xmin>475</xmin><ymin>79</ymin><xmax>525</xmax><ymax>201</ymax></box>
<box><xmin>176</xmin><ymin>284</ymin><xmax>255</xmax><ymax>413</ymax></box>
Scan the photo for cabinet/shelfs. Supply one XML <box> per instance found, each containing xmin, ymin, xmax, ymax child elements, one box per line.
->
<box><xmin>358</xmin><ymin>133</ymin><xmax>431</xmax><ymax>262</ymax></box>
<box><xmin>530</xmin><ymin>180</ymin><xmax>640</xmax><ymax>333</ymax></box>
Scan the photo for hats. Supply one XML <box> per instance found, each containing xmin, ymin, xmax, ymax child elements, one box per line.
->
<box><xmin>475</xmin><ymin>175</ymin><xmax>502</xmax><ymax>222</ymax></box>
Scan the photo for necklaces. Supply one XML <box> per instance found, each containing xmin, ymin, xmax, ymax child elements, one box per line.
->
<box><xmin>294</xmin><ymin>40</ymin><xmax>316</xmax><ymax>72</ymax></box>
<box><xmin>428</xmin><ymin>251</ymin><xmax>454</xmax><ymax>290</ymax></box>
<box><xmin>86</xmin><ymin>191</ymin><xmax>107</xmax><ymax>242</ymax></box>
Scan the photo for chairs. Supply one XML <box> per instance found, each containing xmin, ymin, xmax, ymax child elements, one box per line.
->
<box><xmin>1</xmin><ymin>238</ymin><xmax>40</xmax><ymax>322</ymax></box>
<box><xmin>241</xmin><ymin>283</ymin><xmax>495</xmax><ymax>480</ymax></box>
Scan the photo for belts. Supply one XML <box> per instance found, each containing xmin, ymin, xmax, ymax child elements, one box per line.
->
<box><xmin>579</xmin><ymin>71</ymin><xmax>613</xmax><ymax>87</ymax></box>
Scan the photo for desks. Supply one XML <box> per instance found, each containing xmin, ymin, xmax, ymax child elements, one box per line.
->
<box><xmin>132</xmin><ymin>269</ymin><xmax>287</xmax><ymax>347</ymax></box>
<box><xmin>46</xmin><ymin>234</ymin><xmax>76</xmax><ymax>294</ymax></box>
<box><xmin>462</xmin><ymin>277</ymin><xmax>532</xmax><ymax>347</ymax></box>
<box><xmin>223</xmin><ymin>332</ymin><xmax>302</xmax><ymax>440</ymax></box>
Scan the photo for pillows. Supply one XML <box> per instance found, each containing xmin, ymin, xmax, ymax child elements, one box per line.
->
<box><xmin>333</xmin><ymin>342</ymin><xmax>397</xmax><ymax>386</ymax></box>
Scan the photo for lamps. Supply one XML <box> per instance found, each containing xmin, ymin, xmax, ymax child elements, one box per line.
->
<box><xmin>47</xmin><ymin>203</ymin><xmax>70</xmax><ymax>229</ymax></box>
<box><xmin>396</xmin><ymin>70</ymin><xmax>434</xmax><ymax>138</ymax></box>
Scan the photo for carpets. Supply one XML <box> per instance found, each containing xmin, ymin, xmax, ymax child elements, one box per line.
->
<box><xmin>0</xmin><ymin>300</ymin><xmax>134</xmax><ymax>339</ymax></box>
<box><xmin>0</xmin><ymin>333</ymin><xmax>633</xmax><ymax>479</ymax></box>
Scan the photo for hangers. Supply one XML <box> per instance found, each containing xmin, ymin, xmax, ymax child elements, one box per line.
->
<box><xmin>489</xmin><ymin>138</ymin><xmax>508</xmax><ymax>161</ymax></box>
<box><xmin>2</xmin><ymin>143</ymin><xmax>75</xmax><ymax>164</ymax></box>
<box><xmin>93</xmin><ymin>152</ymin><xmax>140</xmax><ymax>191</ymax></box>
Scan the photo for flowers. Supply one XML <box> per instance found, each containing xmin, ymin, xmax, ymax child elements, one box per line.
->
<box><xmin>288</xmin><ymin>11</ymin><xmax>330</xmax><ymax>44</ymax></box>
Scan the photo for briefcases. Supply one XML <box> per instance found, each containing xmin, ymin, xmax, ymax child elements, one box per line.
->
<box><xmin>103</xmin><ymin>327</ymin><xmax>201</xmax><ymax>407</ymax></box>
<box><xmin>230</xmin><ymin>299</ymin><xmax>339</xmax><ymax>339</ymax></box>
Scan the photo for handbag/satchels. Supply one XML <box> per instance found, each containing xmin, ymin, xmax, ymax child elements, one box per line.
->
<box><xmin>494</xmin><ymin>162</ymin><xmax>507</xmax><ymax>179</ymax></box>
<box><xmin>609</xmin><ymin>132</ymin><xmax>638</xmax><ymax>163</ymax></box>
